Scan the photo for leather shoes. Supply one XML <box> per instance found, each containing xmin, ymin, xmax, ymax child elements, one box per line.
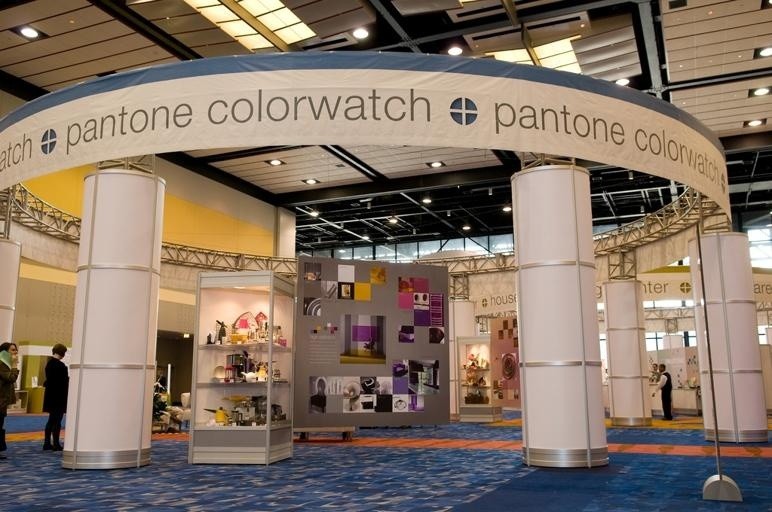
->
<box><xmin>43</xmin><ymin>443</ymin><xmax>62</xmax><ymax>451</ymax></box>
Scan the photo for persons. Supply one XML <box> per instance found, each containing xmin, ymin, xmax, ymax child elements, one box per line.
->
<box><xmin>42</xmin><ymin>343</ymin><xmax>67</xmax><ymax>451</ymax></box>
<box><xmin>649</xmin><ymin>363</ymin><xmax>660</xmax><ymax>382</ymax></box>
<box><xmin>0</xmin><ymin>342</ymin><xmax>19</xmax><ymax>451</ymax></box>
<box><xmin>659</xmin><ymin>366</ymin><xmax>673</xmax><ymax>421</ymax></box>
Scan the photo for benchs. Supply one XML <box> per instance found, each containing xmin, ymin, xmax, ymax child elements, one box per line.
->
<box><xmin>293</xmin><ymin>426</ymin><xmax>356</xmax><ymax>444</ymax></box>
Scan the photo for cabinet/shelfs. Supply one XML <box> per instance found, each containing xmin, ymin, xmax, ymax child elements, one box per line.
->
<box><xmin>187</xmin><ymin>270</ymin><xmax>297</xmax><ymax>466</ymax></box>
<box><xmin>455</xmin><ymin>335</ymin><xmax>503</xmax><ymax>423</ymax></box>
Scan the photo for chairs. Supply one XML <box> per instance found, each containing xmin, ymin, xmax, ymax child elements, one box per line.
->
<box><xmin>153</xmin><ymin>392</ymin><xmax>191</xmax><ymax>434</ymax></box>
